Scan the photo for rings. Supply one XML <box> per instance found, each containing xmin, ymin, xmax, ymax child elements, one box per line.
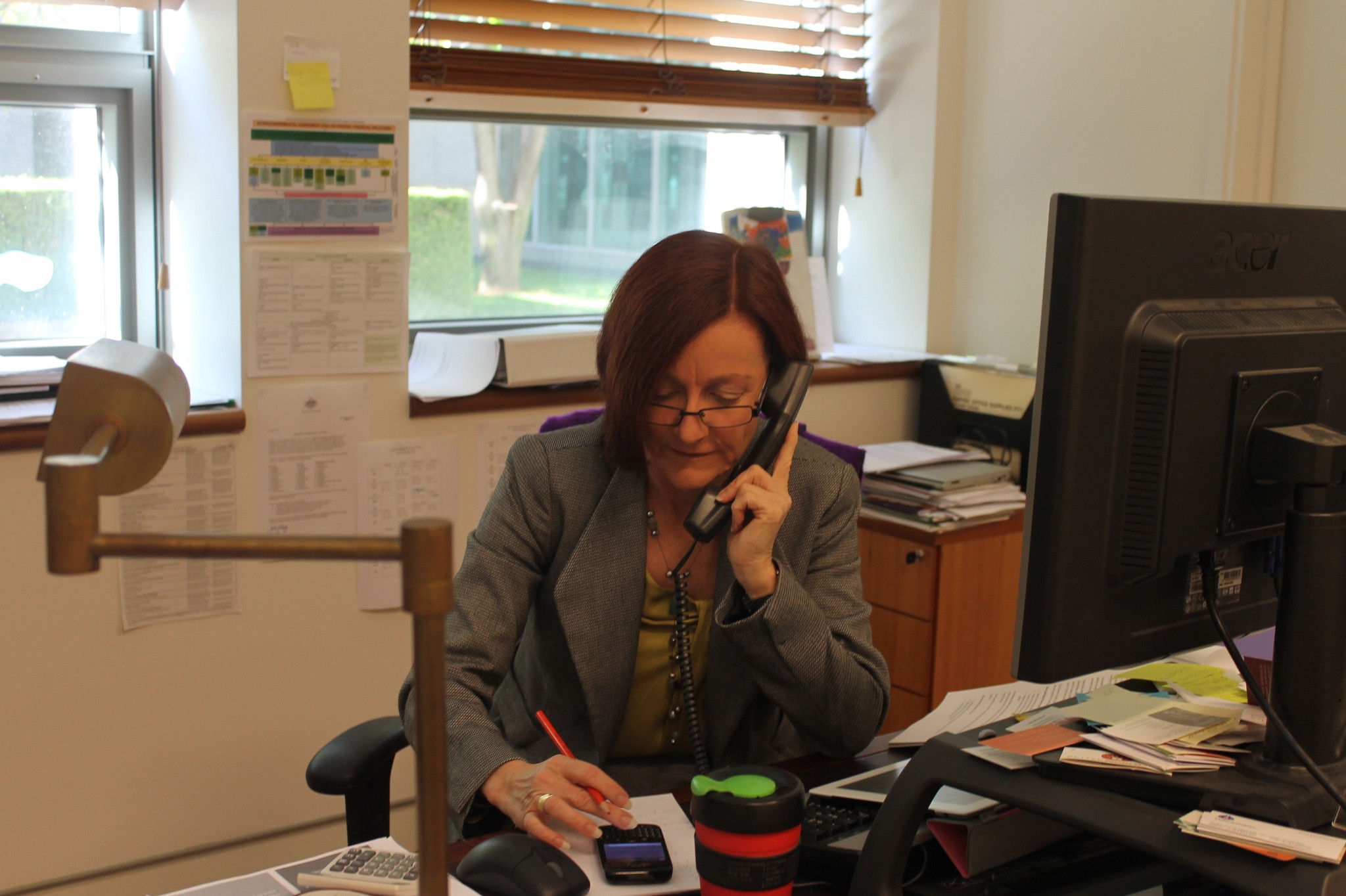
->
<box><xmin>538</xmin><ymin>793</ymin><xmax>553</xmax><ymax>814</ymax></box>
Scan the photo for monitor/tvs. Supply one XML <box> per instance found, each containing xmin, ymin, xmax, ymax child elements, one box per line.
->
<box><xmin>1009</xmin><ymin>192</ymin><xmax>1346</xmax><ymax>829</ymax></box>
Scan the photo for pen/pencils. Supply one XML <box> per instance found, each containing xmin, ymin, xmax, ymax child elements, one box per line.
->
<box><xmin>535</xmin><ymin>709</ymin><xmax>611</xmax><ymax>815</ymax></box>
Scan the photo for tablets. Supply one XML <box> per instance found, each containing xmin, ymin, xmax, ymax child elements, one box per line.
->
<box><xmin>808</xmin><ymin>757</ymin><xmax>998</xmax><ymax>816</ymax></box>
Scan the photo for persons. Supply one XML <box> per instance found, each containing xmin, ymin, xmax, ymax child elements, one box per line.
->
<box><xmin>389</xmin><ymin>225</ymin><xmax>892</xmax><ymax>851</ymax></box>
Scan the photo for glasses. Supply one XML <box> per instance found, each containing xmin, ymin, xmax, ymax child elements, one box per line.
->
<box><xmin>644</xmin><ymin>362</ymin><xmax>776</xmax><ymax>429</ymax></box>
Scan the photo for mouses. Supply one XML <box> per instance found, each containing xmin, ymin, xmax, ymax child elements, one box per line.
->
<box><xmin>455</xmin><ymin>832</ymin><xmax>591</xmax><ymax>896</ymax></box>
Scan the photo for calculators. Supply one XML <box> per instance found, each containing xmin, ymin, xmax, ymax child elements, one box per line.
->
<box><xmin>296</xmin><ymin>846</ymin><xmax>419</xmax><ymax>896</ymax></box>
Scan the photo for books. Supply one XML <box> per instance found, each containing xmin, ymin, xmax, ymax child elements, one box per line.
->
<box><xmin>1085</xmin><ymin>703</ymin><xmax>1251</xmax><ymax>774</ymax></box>
<box><xmin>0</xmin><ymin>354</ymin><xmax>68</xmax><ymax>404</ymax></box>
<box><xmin>851</xmin><ymin>437</ymin><xmax>1028</xmax><ymax>533</ymax></box>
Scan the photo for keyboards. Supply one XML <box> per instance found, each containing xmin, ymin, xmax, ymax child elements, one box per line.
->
<box><xmin>797</xmin><ymin>794</ymin><xmax>934</xmax><ymax>856</ymax></box>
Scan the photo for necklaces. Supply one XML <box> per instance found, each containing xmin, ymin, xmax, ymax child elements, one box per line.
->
<box><xmin>642</xmin><ymin>506</ymin><xmax>705</xmax><ymax>762</ymax></box>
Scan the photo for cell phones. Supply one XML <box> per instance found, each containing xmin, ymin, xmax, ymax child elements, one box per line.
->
<box><xmin>597</xmin><ymin>824</ymin><xmax>673</xmax><ymax>885</ymax></box>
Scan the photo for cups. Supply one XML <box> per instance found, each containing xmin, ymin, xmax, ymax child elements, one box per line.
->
<box><xmin>689</xmin><ymin>765</ymin><xmax>804</xmax><ymax>896</ymax></box>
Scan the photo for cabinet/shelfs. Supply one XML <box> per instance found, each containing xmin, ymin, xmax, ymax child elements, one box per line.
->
<box><xmin>857</xmin><ymin>508</ymin><xmax>1026</xmax><ymax>735</ymax></box>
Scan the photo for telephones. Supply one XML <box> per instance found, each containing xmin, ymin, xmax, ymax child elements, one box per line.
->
<box><xmin>682</xmin><ymin>358</ymin><xmax>814</xmax><ymax>543</ymax></box>
